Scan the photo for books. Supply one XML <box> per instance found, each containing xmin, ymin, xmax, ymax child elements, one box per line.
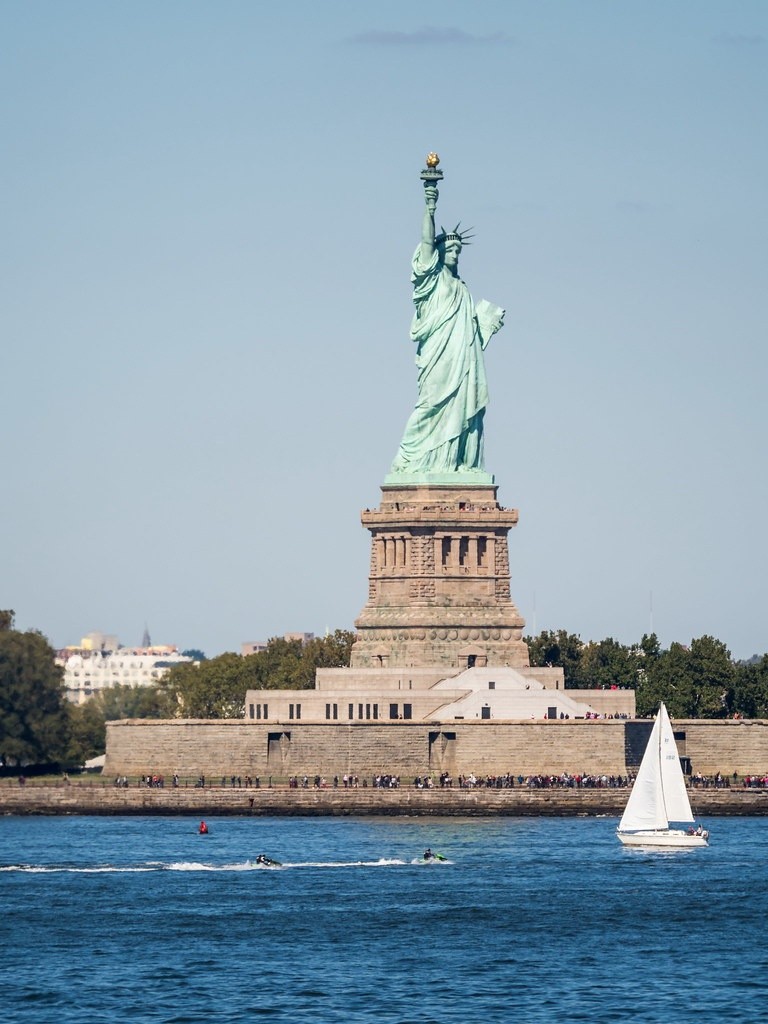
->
<box><xmin>475</xmin><ymin>298</ymin><xmax>505</xmax><ymax>351</ymax></box>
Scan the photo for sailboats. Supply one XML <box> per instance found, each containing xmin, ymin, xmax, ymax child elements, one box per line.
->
<box><xmin>614</xmin><ymin>700</ymin><xmax>710</xmax><ymax>846</ymax></box>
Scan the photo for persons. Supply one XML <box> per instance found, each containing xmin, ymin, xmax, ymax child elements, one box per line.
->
<box><xmin>395</xmin><ymin>713</ymin><xmax>402</xmax><ymax>719</ymax></box>
<box><xmin>172</xmin><ymin>774</ymin><xmax>178</xmax><ymax>788</ymax></box>
<box><xmin>531</xmin><ymin>714</ymin><xmax>535</xmax><ymax>719</ymax></box>
<box><xmin>585</xmin><ymin>711</ymin><xmax>692</xmax><ymax>719</ymax></box>
<box><xmin>199</xmin><ymin>776</ymin><xmax>260</xmax><ymax>788</ymax></box>
<box><xmin>63</xmin><ymin>774</ymin><xmax>68</xmax><ymax>781</ymax></box>
<box><xmin>142</xmin><ymin>775</ymin><xmax>163</xmax><ymax>788</ymax></box>
<box><xmin>412</xmin><ymin>772</ymin><xmax>768</xmax><ymax>788</ymax></box>
<box><xmin>391</xmin><ymin>187</ymin><xmax>504</xmax><ymax>471</ymax></box>
<box><xmin>544</xmin><ymin>712</ymin><xmax>569</xmax><ymax>719</ymax></box>
<box><xmin>727</xmin><ymin>712</ymin><xmax>743</xmax><ymax>719</ymax></box>
<box><xmin>689</xmin><ymin>825</ymin><xmax>701</xmax><ymax>836</ymax></box>
<box><xmin>18</xmin><ymin>775</ymin><xmax>24</xmax><ymax>786</ymax></box>
<box><xmin>290</xmin><ymin>776</ymin><xmax>367</xmax><ymax>788</ymax></box>
<box><xmin>611</xmin><ymin>683</ymin><xmax>625</xmax><ymax>690</ymax></box>
<box><xmin>373</xmin><ymin>774</ymin><xmax>400</xmax><ymax>789</ymax></box>
<box><xmin>256</xmin><ymin>855</ymin><xmax>265</xmax><ymax>864</ymax></box>
<box><xmin>115</xmin><ymin>773</ymin><xmax>127</xmax><ymax>787</ymax></box>
<box><xmin>424</xmin><ymin>849</ymin><xmax>434</xmax><ymax>861</ymax></box>
